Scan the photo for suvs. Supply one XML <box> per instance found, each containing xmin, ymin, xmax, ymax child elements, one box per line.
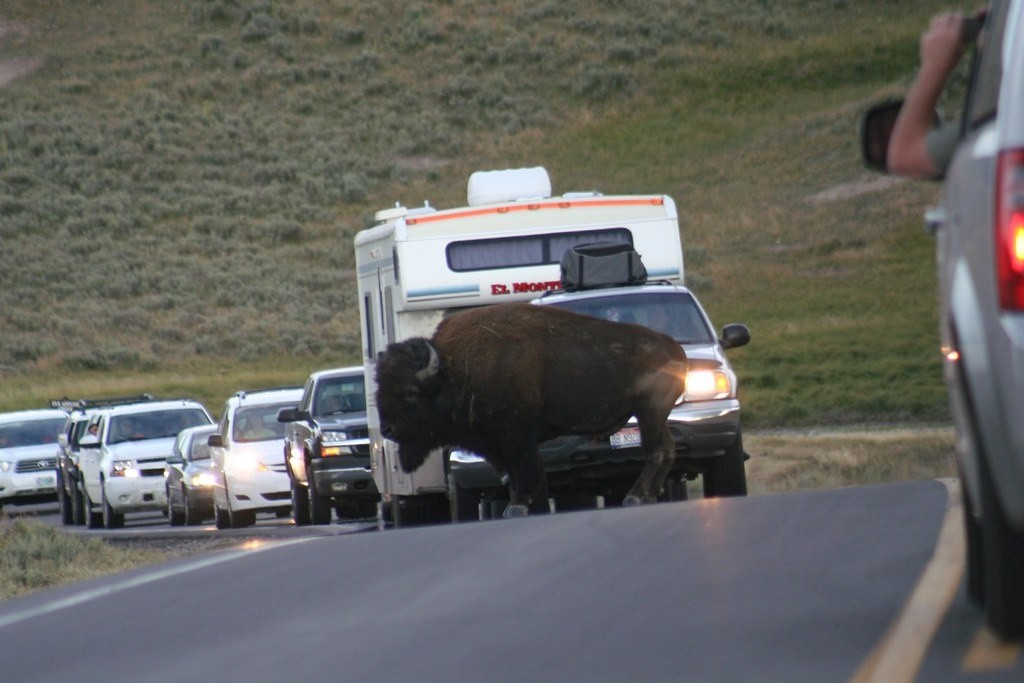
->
<box><xmin>524</xmin><ymin>283</ymin><xmax>749</xmax><ymax>513</ymax></box>
<box><xmin>51</xmin><ymin>392</ymin><xmax>217</xmax><ymax>530</ymax></box>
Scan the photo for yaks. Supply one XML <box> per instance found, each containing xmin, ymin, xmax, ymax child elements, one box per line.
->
<box><xmin>374</xmin><ymin>302</ymin><xmax>688</xmax><ymax>518</ymax></box>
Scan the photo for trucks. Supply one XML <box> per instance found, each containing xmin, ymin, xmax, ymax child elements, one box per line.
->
<box><xmin>353</xmin><ymin>166</ymin><xmax>686</xmax><ymax>530</ymax></box>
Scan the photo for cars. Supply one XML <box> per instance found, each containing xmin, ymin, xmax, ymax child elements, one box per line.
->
<box><xmin>855</xmin><ymin>1</ymin><xmax>1024</xmax><ymax>653</ymax></box>
<box><xmin>0</xmin><ymin>408</ymin><xmax>69</xmax><ymax>510</ymax></box>
<box><xmin>277</xmin><ymin>364</ymin><xmax>368</xmax><ymax>528</ymax></box>
<box><xmin>162</xmin><ymin>422</ymin><xmax>217</xmax><ymax>528</ymax></box>
<box><xmin>207</xmin><ymin>385</ymin><xmax>307</xmax><ymax>530</ymax></box>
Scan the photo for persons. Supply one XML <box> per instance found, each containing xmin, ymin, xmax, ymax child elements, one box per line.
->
<box><xmin>646</xmin><ymin>303</ymin><xmax>682</xmax><ymax>338</ymax></box>
<box><xmin>321</xmin><ymin>386</ymin><xmax>354</xmax><ymax>415</ymax></box>
<box><xmin>119</xmin><ymin>419</ymin><xmax>145</xmax><ymax>438</ymax></box>
<box><xmin>89</xmin><ymin>425</ymin><xmax>98</xmax><ymax>436</ymax></box>
<box><xmin>0</xmin><ymin>431</ymin><xmax>13</xmax><ymax>447</ymax></box>
<box><xmin>178</xmin><ymin>413</ymin><xmax>192</xmax><ymax>429</ymax></box>
<box><xmin>881</xmin><ymin>4</ymin><xmax>994</xmax><ymax>181</ymax></box>
<box><xmin>244</xmin><ymin>414</ymin><xmax>277</xmax><ymax>441</ymax></box>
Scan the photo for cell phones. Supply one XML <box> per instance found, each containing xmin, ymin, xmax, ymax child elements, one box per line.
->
<box><xmin>962</xmin><ymin>18</ymin><xmax>984</xmax><ymax>43</ymax></box>
